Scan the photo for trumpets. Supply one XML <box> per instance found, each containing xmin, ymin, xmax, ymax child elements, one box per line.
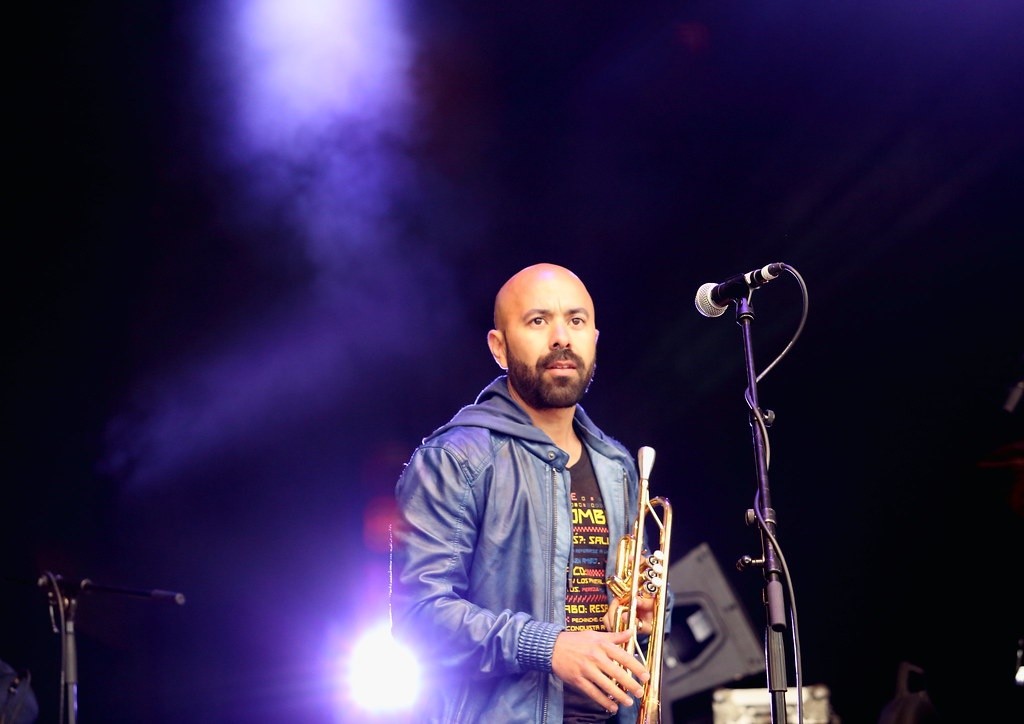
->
<box><xmin>603</xmin><ymin>445</ymin><xmax>676</xmax><ymax>723</ymax></box>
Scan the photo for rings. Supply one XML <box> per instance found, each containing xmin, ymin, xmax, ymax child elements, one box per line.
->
<box><xmin>636</xmin><ymin>618</ymin><xmax>643</xmax><ymax>632</ymax></box>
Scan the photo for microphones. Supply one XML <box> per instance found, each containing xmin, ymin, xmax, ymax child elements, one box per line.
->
<box><xmin>695</xmin><ymin>262</ymin><xmax>786</xmax><ymax>318</ymax></box>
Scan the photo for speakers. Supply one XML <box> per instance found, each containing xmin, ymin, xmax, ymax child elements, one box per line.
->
<box><xmin>660</xmin><ymin>542</ymin><xmax>769</xmax><ymax>703</ymax></box>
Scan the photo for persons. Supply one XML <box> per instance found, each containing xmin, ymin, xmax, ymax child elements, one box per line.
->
<box><xmin>390</xmin><ymin>263</ymin><xmax>676</xmax><ymax>724</ymax></box>
